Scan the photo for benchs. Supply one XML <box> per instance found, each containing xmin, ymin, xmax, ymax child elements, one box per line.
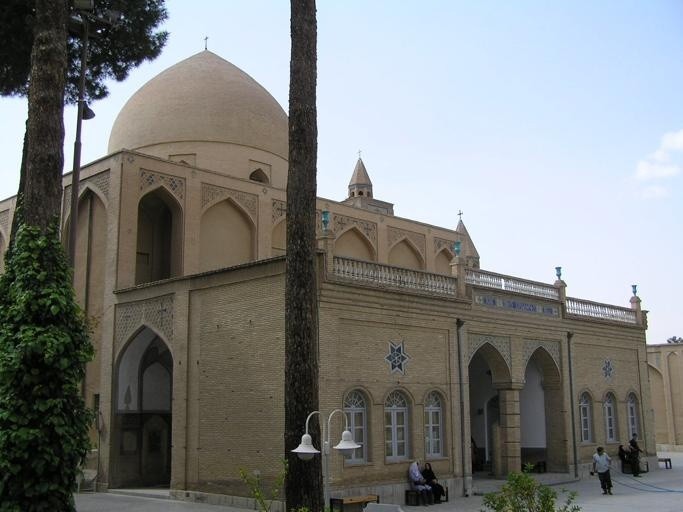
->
<box><xmin>405</xmin><ymin>485</ymin><xmax>448</xmax><ymax>506</ymax></box>
<box><xmin>622</xmin><ymin>461</ymin><xmax>648</xmax><ymax>473</ymax></box>
<box><xmin>657</xmin><ymin>457</ymin><xmax>672</xmax><ymax>469</ymax></box>
<box><xmin>329</xmin><ymin>494</ymin><xmax>379</xmax><ymax>512</ymax></box>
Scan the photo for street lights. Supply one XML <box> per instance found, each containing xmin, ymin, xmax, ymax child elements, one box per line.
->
<box><xmin>292</xmin><ymin>409</ymin><xmax>362</xmax><ymax>511</ymax></box>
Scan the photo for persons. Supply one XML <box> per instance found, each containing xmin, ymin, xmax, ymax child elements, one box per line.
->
<box><xmin>590</xmin><ymin>447</ymin><xmax>613</xmax><ymax>495</ymax></box>
<box><xmin>619</xmin><ymin>433</ymin><xmax>644</xmax><ymax>477</ymax></box>
<box><xmin>409</xmin><ymin>461</ymin><xmax>442</xmax><ymax>506</ymax></box>
